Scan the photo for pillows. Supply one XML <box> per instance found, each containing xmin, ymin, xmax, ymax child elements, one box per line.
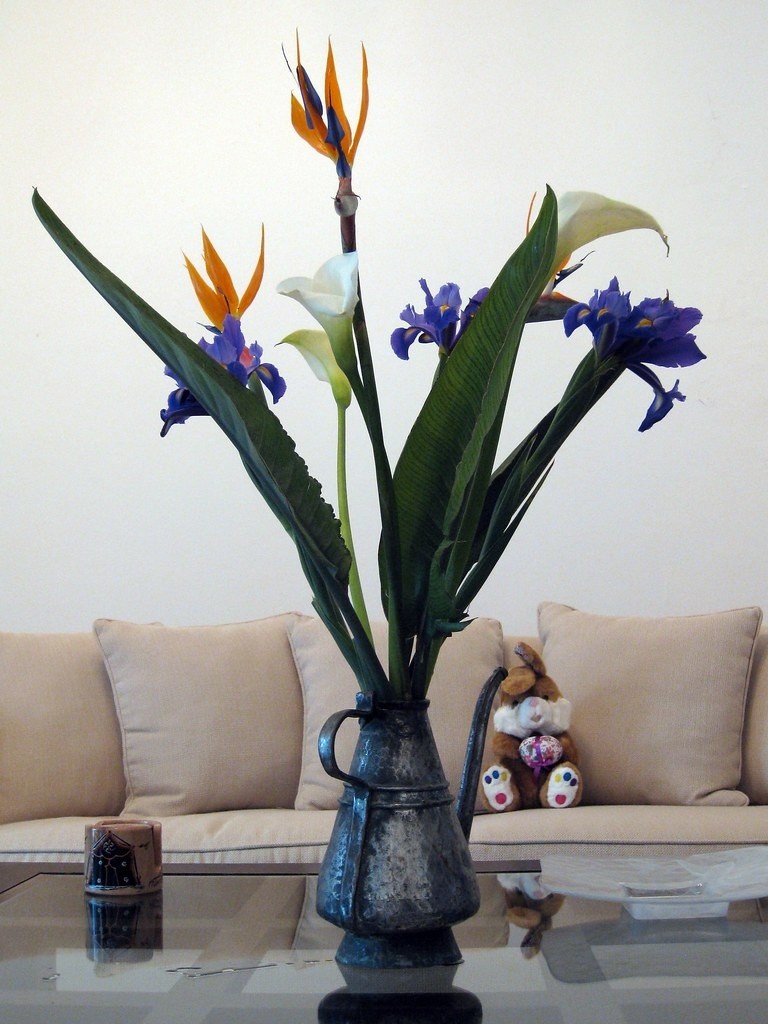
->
<box><xmin>536</xmin><ymin>603</ymin><xmax>763</xmax><ymax>807</ymax></box>
<box><xmin>283</xmin><ymin>614</ymin><xmax>502</xmax><ymax>813</ymax></box>
<box><xmin>0</xmin><ymin>621</ymin><xmax>165</xmax><ymax>825</ymax></box>
<box><xmin>92</xmin><ymin>612</ymin><xmax>303</xmax><ymax>818</ymax></box>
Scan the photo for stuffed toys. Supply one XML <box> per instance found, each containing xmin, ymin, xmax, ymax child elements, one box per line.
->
<box><xmin>496</xmin><ymin>873</ymin><xmax>566</xmax><ymax>961</ymax></box>
<box><xmin>480</xmin><ymin>639</ymin><xmax>583</xmax><ymax>813</ymax></box>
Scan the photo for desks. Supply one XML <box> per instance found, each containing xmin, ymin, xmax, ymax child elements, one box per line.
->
<box><xmin>0</xmin><ymin>861</ymin><xmax>768</xmax><ymax>1024</ymax></box>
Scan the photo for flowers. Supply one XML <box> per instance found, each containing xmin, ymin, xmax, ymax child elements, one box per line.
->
<box><xmin>32</xmin><ymin>27</ymin><xmax>709</xmax><ymax>697</ymax></box>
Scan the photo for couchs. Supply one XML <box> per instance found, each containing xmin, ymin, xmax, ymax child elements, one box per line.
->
<box><xmin>0</xmin><ymin>636</ymin><xmax>768</xmax><ymax>863</ymax></box>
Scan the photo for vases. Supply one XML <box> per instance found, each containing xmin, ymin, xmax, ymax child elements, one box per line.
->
<box><xmin>316</xmin><ymin>667</ymin><xmax>509</xmax><ymax>1024</ymax></box>
